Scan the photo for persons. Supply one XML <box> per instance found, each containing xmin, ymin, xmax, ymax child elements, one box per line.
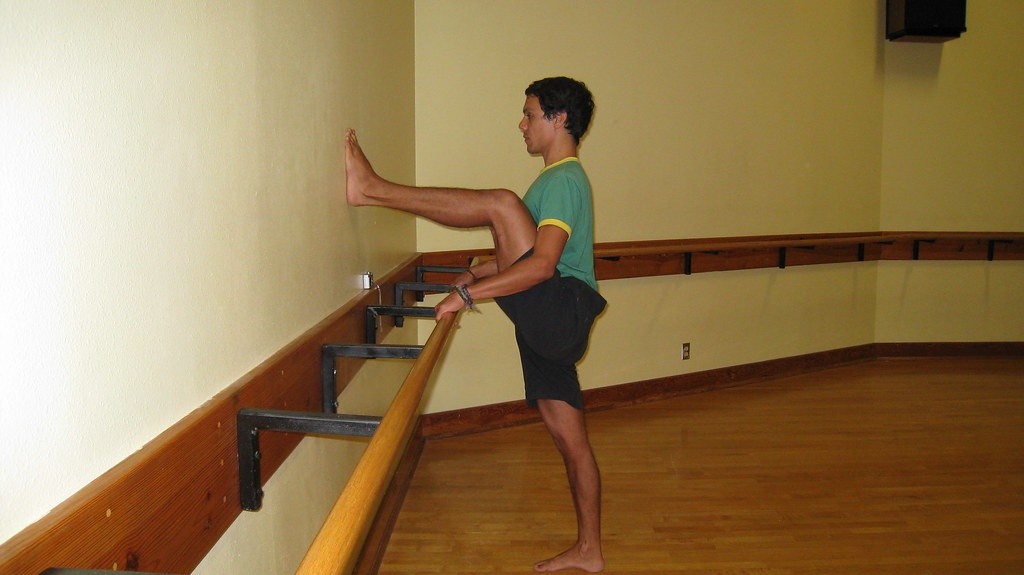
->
<box><xmin>343</xmin><ymin>77</ymin><xmax>605</xmax><ymax>573</ymax></box>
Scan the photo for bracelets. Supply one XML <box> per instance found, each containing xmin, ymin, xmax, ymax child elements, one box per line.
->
<box><xmin>464</xmin><ymin>269</ymin><xmax>476</xmax><ymax>279</ymax></box>
<box><xmin>454</xmin><ymin>284</ymin><xmax>474</xmax><ymax>310</ymax></box>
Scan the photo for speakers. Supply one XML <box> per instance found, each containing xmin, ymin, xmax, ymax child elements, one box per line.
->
<box><xmin>884</xmin><ymin>0</ymin><xmax>968</xmax><ymax>44</ymax></box>
